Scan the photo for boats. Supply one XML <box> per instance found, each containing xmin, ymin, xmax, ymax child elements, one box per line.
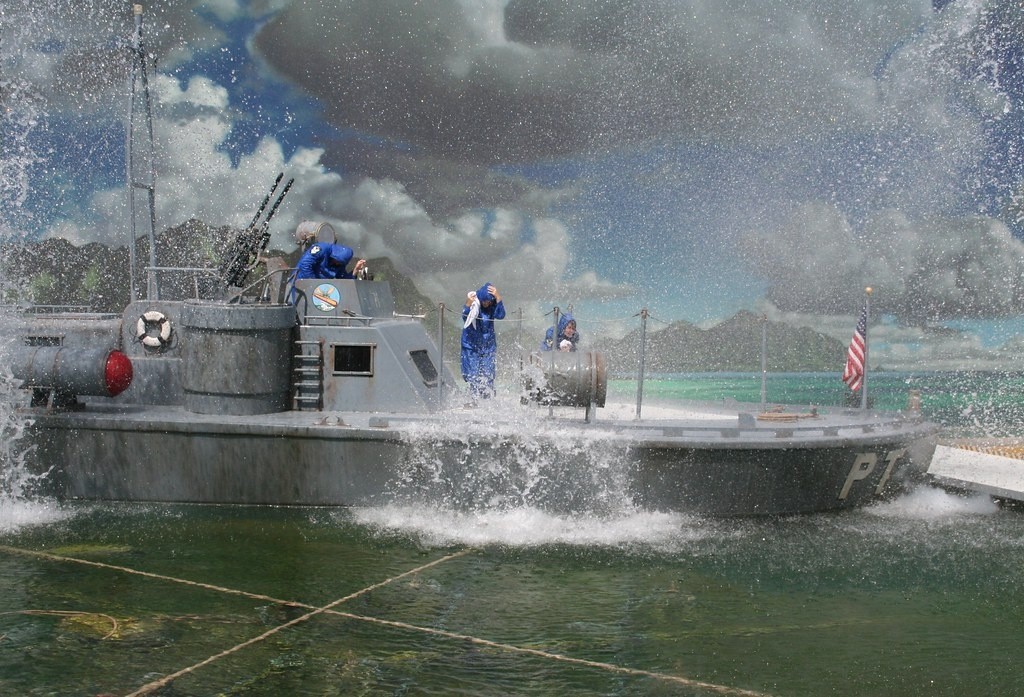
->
<box><xmin>0</xmin><ymin>1</ymin><xmax>940</xmax><ymax>513</ymax></box>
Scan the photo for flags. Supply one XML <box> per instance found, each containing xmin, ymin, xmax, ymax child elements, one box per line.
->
<box><xmin>843</xmin><ymin>300</ymin><xmax>866</xmax><ymax>392</ymax></box>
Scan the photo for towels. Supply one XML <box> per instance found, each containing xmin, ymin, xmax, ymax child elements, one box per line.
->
<box><xmin>463</xmin><ymin>291</ymin><xmax>481</xmax><ymax>330</ymax></box>
<box><xmin>559</xmin><ymin>339</ymin><xmax>574</xmax><ymax>349</ymax></box>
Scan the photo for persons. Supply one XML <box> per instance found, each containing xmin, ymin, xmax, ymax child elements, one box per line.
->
<box><xmin>541</xmin><ymin>313</ymin><xmax>580</xmax><ymax>352</ymax></box>
<box><xmin>460</xmin><ymin>282</ymin><xmax>506</xmax><ymax>399</ymax></box>
<box><xmin>289</xmin><ymin>242</ymin><xmax>367</xmax><ymax>306</ymax></box>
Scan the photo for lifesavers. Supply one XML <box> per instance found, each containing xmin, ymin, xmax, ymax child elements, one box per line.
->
<box><xmin>137</xmin><ymin>311</ymin><xmax>171</xmax><ymax>347</ymax></box>
